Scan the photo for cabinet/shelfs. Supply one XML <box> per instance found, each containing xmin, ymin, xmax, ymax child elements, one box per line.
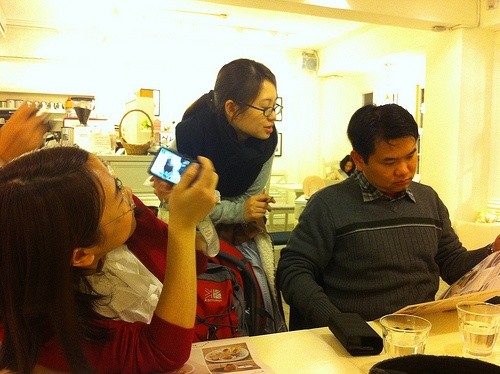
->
<box><xmin>94</xmin><ymin>155</ymin><xmax>171</xmax><ymax>230</ymax></box>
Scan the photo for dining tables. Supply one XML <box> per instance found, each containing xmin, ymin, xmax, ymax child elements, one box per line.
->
<box><xmin>0</xmin><ymin>301</ymin><xmax>500</xmax><ymax>374</ymax></box>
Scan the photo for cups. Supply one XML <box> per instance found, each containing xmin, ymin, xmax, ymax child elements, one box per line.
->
<box><xmin>379</xmin><ymin>314</ymin><xmax>432</xmax><ymax>358</ymax></box>
<box><xmin>456</xmin><ymin>300</ymin><xmax>500</xmax><ymax>358</ymax></box>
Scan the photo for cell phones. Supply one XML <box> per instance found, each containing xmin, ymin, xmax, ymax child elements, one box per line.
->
<box><xmin>147</xmin><ymin>147</ymin><xmax>202</xmax><ymax>186</ymax></box>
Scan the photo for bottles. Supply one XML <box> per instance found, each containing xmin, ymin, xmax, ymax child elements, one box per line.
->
<box><xmin>65</xmin><ymin>96</ymin><xmax>73</xmax><ymax>117</ymax></box>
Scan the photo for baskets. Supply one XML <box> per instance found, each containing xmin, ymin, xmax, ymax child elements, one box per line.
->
<box><xmin>119</xmin><ymin>109</ymin><xmax>154</xmax><ymax>155</ymax></box>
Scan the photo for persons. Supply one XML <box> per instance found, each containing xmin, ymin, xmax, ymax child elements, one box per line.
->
<box><xmin>276</xmin><ymin>104</ymin><xmax>500</xmax><ymax>329</ymax></box>
<box><xmin>157</xmin><ymin>58</ymin><xmax>283</xmax><ymax>225</ymax></box>
<box><xmin>179</xmin><ymin>158</ymin><xmax>190</xmax><ymax>175</ymax></box>
<box><xmin>326</xmin><ymin>155</ymin><xmax>357</xmax><ymax>186</ymax></box>
<box><xmin>1</xmin><ymin>147</ymin><xmax>218</xmax><ymax>374</ymax></box>
<box><xmin>164</xmin><ymin>158</ymin><xmax>174</xmax><ymax>172</ymax></box>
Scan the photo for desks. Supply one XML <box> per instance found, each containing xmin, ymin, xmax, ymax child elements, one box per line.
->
<box><xmin>267</xmin><ymin>203</ymin><xmax>308</xmax><ymax>231</ymax></box>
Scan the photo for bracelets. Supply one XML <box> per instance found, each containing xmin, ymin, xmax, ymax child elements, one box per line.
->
<box><xmin>489</xmin><ymin>242</ymin><xmax>495</xmax><ymax>253</ymax></box>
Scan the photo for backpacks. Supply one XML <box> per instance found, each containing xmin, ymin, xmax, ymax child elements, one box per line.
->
<box><xmin>191</xmin><ymin>252</ymin><xmax>277</xmax><ymax>344</ymax></box>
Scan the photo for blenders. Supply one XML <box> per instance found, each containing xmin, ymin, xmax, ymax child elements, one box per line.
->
<box><xmin>70</xmin><ymin>97</ymin><xmax>103</xmax><ymax>154</ymax></box>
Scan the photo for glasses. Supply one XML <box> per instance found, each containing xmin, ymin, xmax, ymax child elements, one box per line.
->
<box><xmin>230</xmin><ymin>96</ymin><xmax>283</xmax><ymax>117</ymax></box>
<box><xmin>84</xmin><ymin>159</ymin><xmax>136</xmax><ymax>235</ymax></box>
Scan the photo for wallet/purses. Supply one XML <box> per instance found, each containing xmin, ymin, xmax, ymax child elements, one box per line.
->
<box><xmin>328</xmin><ymin>312</ymin><xmax>384</xmax><ymax>357</ymax></box>
